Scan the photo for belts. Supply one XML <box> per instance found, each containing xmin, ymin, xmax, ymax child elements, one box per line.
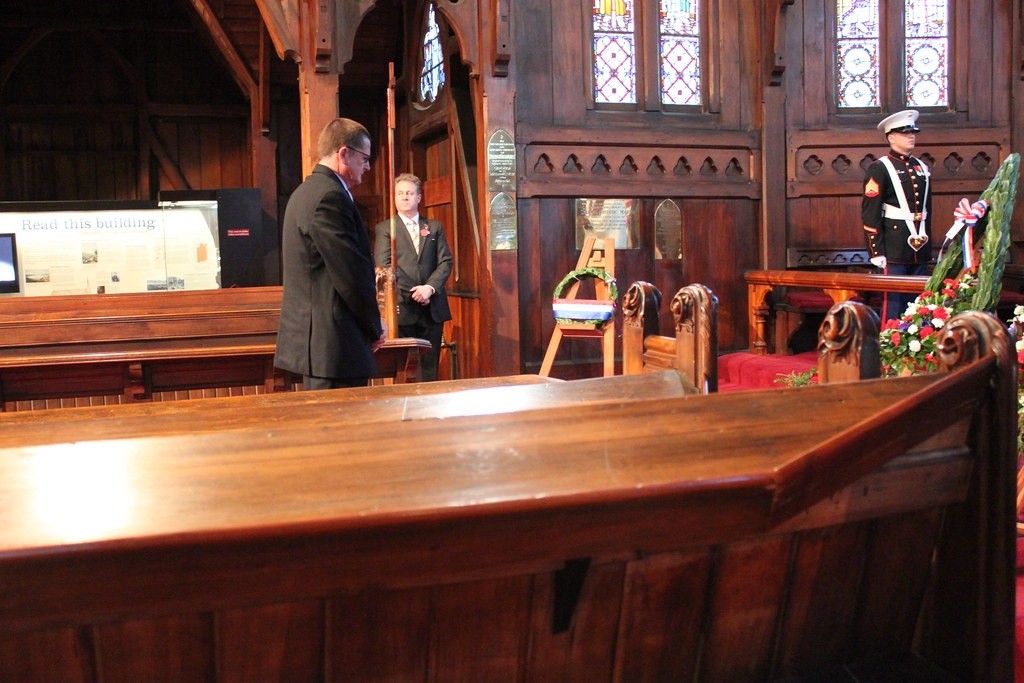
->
<box><xmin>881</xmin><ymin>203</ymin><xmax>928</xmax><ymax>221</ymax></box>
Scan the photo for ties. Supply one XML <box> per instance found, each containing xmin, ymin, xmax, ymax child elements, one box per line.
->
<box><xmin>408</xmin><ymin>219</ymin><xmax>419</xmax><ymax>255</ymax></box>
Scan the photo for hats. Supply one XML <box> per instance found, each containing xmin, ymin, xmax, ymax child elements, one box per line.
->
<box><xmin>876</xmin><ymin>109</ymin><xmax>920</xmax><ymax>134</ymax></box>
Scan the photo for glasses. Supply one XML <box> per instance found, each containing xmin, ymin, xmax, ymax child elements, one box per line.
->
<box><xmin>336</xmin><ymin>146</ymin><xmax>371</xmax><ymax>164</ymax></box>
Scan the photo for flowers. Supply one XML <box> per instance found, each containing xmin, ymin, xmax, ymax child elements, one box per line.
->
<box><xmin>777</xmin><ymin>275</ymin><xmax>964</xmax><ymax>387</ymax></box>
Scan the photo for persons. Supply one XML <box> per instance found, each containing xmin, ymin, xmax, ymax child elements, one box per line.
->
<box><xmin>373</xmin><ymin>174</ymin><xmax>453</xmax><ymax>382</ymax></box>
<box><xmin>274</xmin><ymin>117</ymin><xmax>386</xmax><ymax>390</ymax></box>
<box><xmin>861</xmin><ymin>110</ymin><xmax>931</xmax><ymax>320</ymax></box>
